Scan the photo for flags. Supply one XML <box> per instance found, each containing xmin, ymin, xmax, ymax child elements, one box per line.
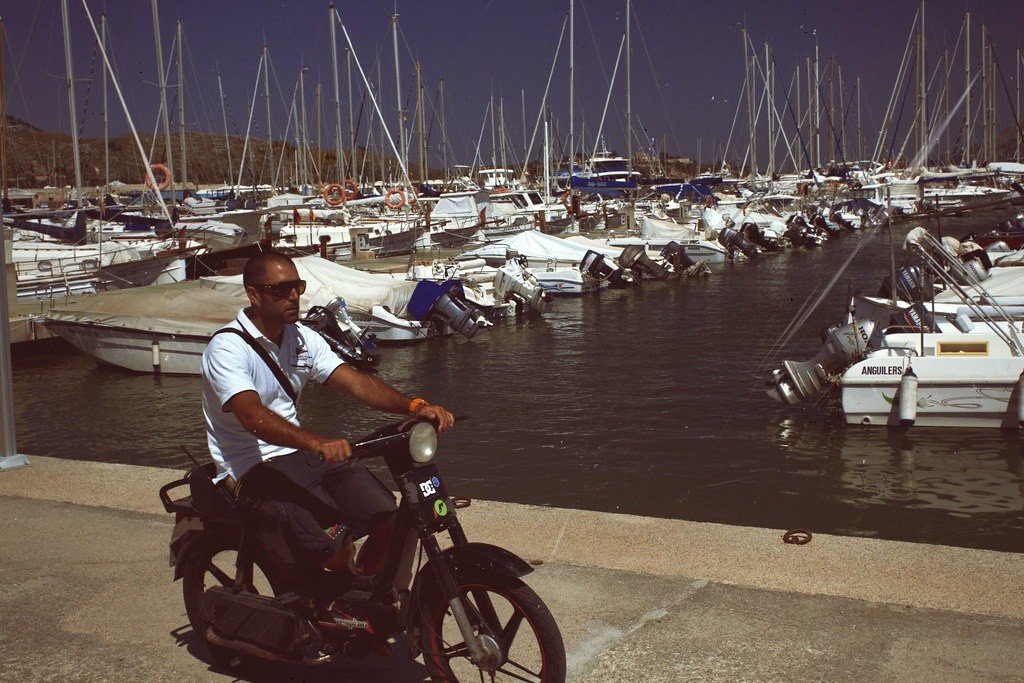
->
<box><xmin>294</xmin><ymin>208</ymin><xmax>301</xmax><ymax>226</ymax></box>
<box><xmin>310</xmin><ymin>207</ymin><xmax>315</xmax><ymax>220</ymax></box>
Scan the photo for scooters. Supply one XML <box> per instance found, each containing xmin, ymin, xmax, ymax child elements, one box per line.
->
<box><xmin>158</xmin><ymin>412</ymin><xmax>568</xmax><ymax>683</ymax></box>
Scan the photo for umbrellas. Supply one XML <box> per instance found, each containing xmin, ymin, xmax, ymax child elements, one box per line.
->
<box><xmin>103</xmin><ymin>180</ymin><xmax>126</xmax><ymax>187</ymax></box>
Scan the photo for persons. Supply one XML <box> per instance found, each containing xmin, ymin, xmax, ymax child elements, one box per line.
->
<box><xmin>201</xmin><ymin>248</ymin><xmax>456</xmax><ymax>634</ymax></box>
<box><xmin>111</xmin><ymin>189</ymin><xmax>116</xmax><ymax>193</ymax></box>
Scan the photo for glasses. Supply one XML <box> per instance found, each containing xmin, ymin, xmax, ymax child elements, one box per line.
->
<box><xmin>245</xmin><ymin>279</ymin><xmax>306</xmax><ymax>298</ymax></box>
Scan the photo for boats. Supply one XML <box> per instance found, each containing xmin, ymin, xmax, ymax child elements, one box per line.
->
<box><xmin>29</xmin><ymin>278</ymin><xmax>380</xmax><ymax>379</ymax></box>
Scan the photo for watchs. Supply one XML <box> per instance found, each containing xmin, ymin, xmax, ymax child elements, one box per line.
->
<box><xmin>409</xmin><ymin>398</ymin><xmax>431</xmax><ymax>415</ymax></box>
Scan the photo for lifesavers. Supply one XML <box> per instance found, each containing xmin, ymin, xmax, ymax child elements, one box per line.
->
<box><xmin>707</xmin><ymin>195</ymin><xmax>715</xmax><ymax>208</ymax></box>
<box><xmin>408</xmin><ymin>186</ymin><xmax>418</xmax><ymax>204</ymax></box>
<box><xmin>324</xmin><ymin>184</ymin><xmax>345</xmax><ymax>205</ymax></box>
<box><xmin>493</xmin><ymin>190</ymin><xmax>512</xmax><ymax>194</ymax></box>
<box><xmin>145</xmin><ymin>164</ymin><xmax>170</xmax><ymax>191</ymax></box>
<box><xmin>492</xmin><ymin>187</ymin><xmax>511</xmax><ymax>190</ymax></box>
<box><xmin>338</xmin><ymin>180</ymin><xmax>358</xmax><ymax>201</ymax></box>
<box><xmin>563</xmin><ymin>191</ymin><xmax>580</xmax><ymax>213</ymax></box>
<box><xmin>386</xmin><ymin>189</ymin><xmax>407</xmax><ymax>208</ymax></box>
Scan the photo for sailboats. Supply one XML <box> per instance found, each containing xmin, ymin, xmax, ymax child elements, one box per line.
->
<box><xmin>0</xmin><ymin>0</ymin><xmax>1024</xmax><ymax>435</ymax></box>
